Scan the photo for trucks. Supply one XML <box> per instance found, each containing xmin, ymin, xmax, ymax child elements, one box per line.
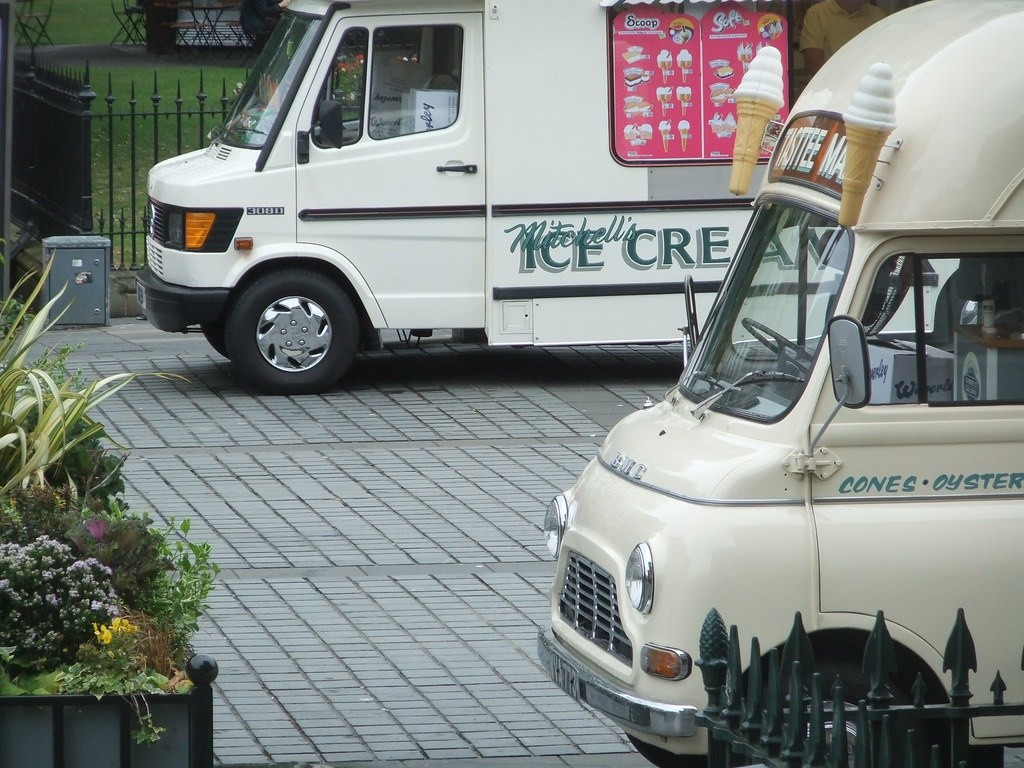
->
<box><xmin>135</xmin><ymin>0</ymin><xmax>960</xmax><ymax>394</ymax></box>
<box><xmin>536</xmin><ymin>0</ymin><xmax>1024</xmax><ymax>768</ymax></box>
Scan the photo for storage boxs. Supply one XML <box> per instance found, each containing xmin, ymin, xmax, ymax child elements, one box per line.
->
<box><xmin>867</xmin><ymin>338</ymin><xmax>954</xmax><ymax>404</ymax></box>
<box><xmin>369</xmin><ymin>52</ymin><xmax>432</xmax><ymax>138</ymax></box>
<box><xmin>400</xmin><ymin>92</ymin><xmax>412</xmax><ymax>135</ymax></box>
<box><xmin>407</xmin><ymin>87</ymin><xmax>457</xmax><ymax>132</ymax></box>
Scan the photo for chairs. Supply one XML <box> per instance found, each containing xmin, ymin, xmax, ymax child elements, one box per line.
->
<box><xmin>396</xmin><ymin>72</ymin><xmax>458</xmax><ymax>127</ymax></box>
<box><xmin>934</xmin><ymin>259</ymin><xmax>997</xmax><ymax>353</ymax></box>
<box><xmin>14</xmin><ymin>0</ymin><xmax>259</xmax><ymax>69</ymax></box>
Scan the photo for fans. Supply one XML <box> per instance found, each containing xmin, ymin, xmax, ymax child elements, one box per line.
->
<box><xmin>862</xmin><ymin>254</ymin><xmax>914</xmax><ymax>336</ymax></box>
